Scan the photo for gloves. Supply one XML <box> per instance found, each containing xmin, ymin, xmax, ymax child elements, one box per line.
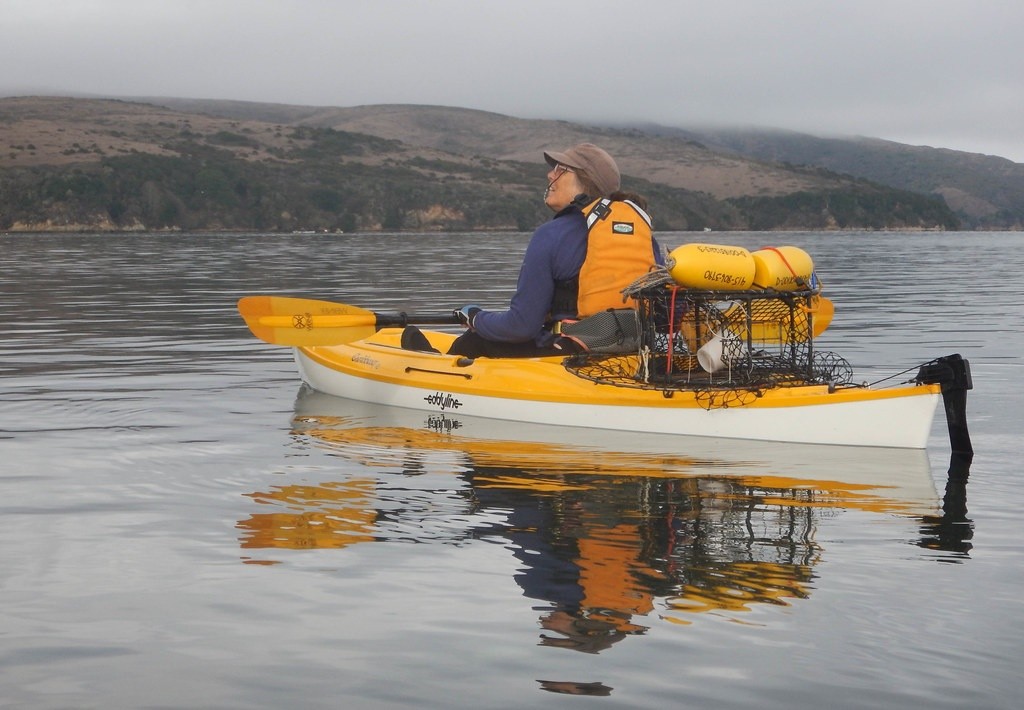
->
<box><xmin>452</xmin><ymin>304</ymin><xmax>482</xmax><ymax>329</ymax></box>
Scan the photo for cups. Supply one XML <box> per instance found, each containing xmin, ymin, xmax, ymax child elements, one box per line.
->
<box><xmin>696</xmin><ymin>328</ymin><xmax>745</xmax><ymax>373</ymax></box>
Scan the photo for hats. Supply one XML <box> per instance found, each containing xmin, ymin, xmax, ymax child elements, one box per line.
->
<box><xmin>543</xmin><ymin>142</ymin><xmax>621</xmax><ymax>197</ymax></box>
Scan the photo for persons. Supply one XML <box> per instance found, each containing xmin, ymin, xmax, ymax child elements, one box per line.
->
<box><xmin>400</xmin><ymin>144</ymin><xmax>687</xmax><ymax>358</ymax></box>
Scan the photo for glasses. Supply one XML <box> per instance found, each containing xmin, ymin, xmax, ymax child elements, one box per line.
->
<box><xmin>554</xmin><ymin>163</ymin><xmax>576</xmax><ymax>176</ymax></box>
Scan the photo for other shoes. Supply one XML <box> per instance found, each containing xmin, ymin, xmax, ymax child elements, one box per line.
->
<box><xmin>401</xmin><ymin>326</ymin><xmax>432</xmax><ymax>351</ymax></box>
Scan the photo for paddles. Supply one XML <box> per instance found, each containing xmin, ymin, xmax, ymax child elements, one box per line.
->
<box><xmin>237</xmin><ymin>293</ymin><xmax>834</xmax><ymax>347</ymax></box>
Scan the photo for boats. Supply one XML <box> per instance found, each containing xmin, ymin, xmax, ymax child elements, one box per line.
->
<box><xmin>291</xmin><ymin>324</ymin><xmax>942</xmax><ymax>450</ymax></box>
<box><xmin>286</xmin><ymin>382</ymin><xmax>950</xmax><ymax>518</ymax></box>
